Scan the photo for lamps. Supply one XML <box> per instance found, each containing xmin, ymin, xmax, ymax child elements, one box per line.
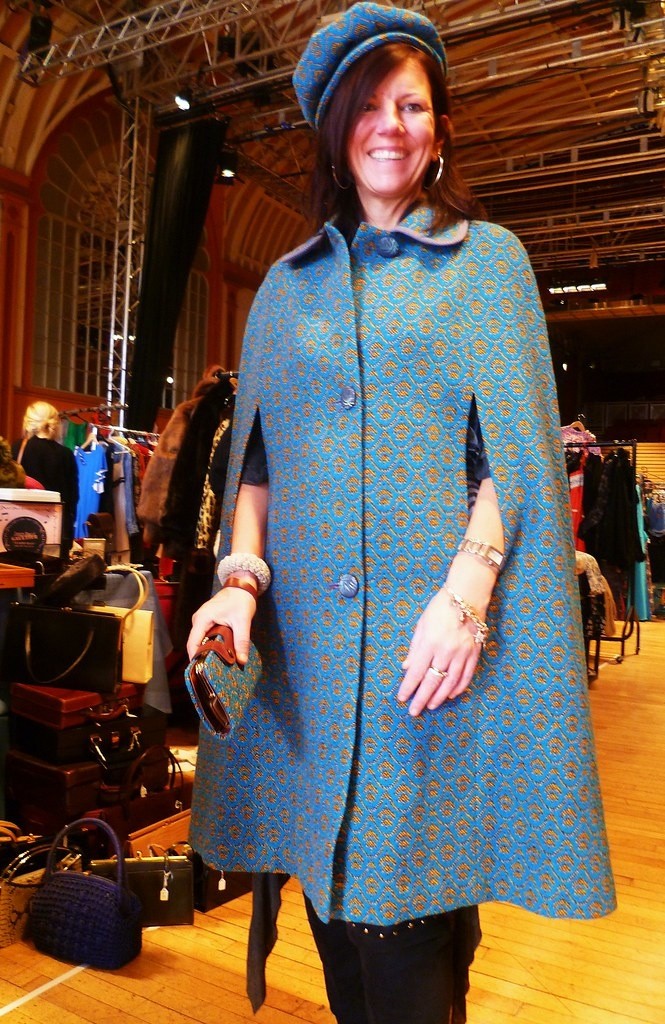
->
<box><xmin>549</xmin><ymin>276</ymin><xmax>607</xmax><ymax>294</ymax></box>
<box><xmin>173</xmin><ymin>87</ymin><xmax>199</xmax><ymax>113</ymax></box>
<box><xmin>220</xmin><ymin>154</ymin><xmax>238</xmax><ymax>178</ymax></box>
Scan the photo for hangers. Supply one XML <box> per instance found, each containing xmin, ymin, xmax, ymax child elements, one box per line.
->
<box><xmin>568</xmin><ymin>412</ymin><xmax>587</xmax><ymax>434</ymax></box>
<box><xmin>563</xmin><ymin>438</ymin><xmax>631</xmax><ymax>463</ymax></box>
<box><xmin>79</xmin><ymin>420</ymin><xmax>161</xmax><ymax>453</ymax></box>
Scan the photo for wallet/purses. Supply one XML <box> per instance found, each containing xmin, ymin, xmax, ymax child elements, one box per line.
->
<box><xmin>184</xmin><ymin>623</ymin><xmax>263</xmax><ymax>739</ymax></box>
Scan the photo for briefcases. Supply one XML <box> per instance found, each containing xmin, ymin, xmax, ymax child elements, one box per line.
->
<box><xmin>8</xmin><ymin>681</ymin><xmax>147</xmax><ymax>730</ymax></box>
<box><xmin>9</xmin><ymin>702</ymin><xmax>166</xmax><ymax>766</ymax></box>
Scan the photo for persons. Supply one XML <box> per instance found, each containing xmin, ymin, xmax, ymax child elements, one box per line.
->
<box><xmin>185</xmin><ymin>1</ymin><xmax>620</xmax><ymax>1024</ymax></box>
<box><xmin>12</xmin><ymin>402</ymin><xmax>79</xmax><ymax>575</ymax></box>
<box><xmin>0</xmin><ymin>436</ymin><xmax>45</xmax><ymax>490</ymax></box>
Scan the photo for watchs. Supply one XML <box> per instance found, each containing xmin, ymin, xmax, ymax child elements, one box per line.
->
<box><xmin>458</xmin><ymin>539</ymin><xmax>504</xmax><ymax>572</ymax></box>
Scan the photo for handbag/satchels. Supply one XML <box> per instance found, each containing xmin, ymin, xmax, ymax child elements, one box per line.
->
<box><xmin>0</xmin><ymin>844</ymin><xmax>82</xmax><ymax>948</ymax></box>
<box><xmin>66</xmin><ymin>565</ymin><xmax>155</xmax><ymax>685</ymax></box>
<box><xmin>30</xmin><ymin>818</ymin><xmax>142</xmax><ymax>971</ymax></box>
<box><xmin>83</xmin><ymin>513</ymin><xmax>115</xmax><ymax>553</ymax></box>
<box><xmin>122</xmin><ymin>808</ymin><xmax>192</xmax><ymax>860</ymax></box>
<box><xmin>82</xmin><ymin>744</ymin><xmax>193</xmax><ymax>858</ymax></box>
<box><xmin>0</xmin><ymin>820</ymin><xmax>46</xmax><ymax>879</ymax></box>
<box><xmin>88</xmin><ymin>854</ymin><xmax>195</xmax><ymax>927</ymax></box>
<box><xmin>194</xmin><ymin>850</ymin><xmax>254</xmax><ymax>912</ymax></box>
<box><xmin>0</xmin><ymin>603</ymin><xmax>126</xmax><ymax>694</ymax></box>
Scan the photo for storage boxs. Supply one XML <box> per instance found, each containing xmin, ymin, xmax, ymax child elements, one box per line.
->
<box><xmin>7</xmin><ymin>682</ymin><xmax>145</xmax><ymax>831</ymax></box>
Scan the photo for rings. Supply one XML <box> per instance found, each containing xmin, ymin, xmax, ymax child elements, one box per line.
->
<box><xmin>429</xmin><ymin>667</ymin><xmax>447</xmax><ymax>677</ymax></box>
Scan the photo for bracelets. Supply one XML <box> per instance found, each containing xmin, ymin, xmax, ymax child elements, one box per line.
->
<box><xmin>222</xmin><ymin>577</ymin><xmax>257</xmax><ymax>603</ymax></box>
<box><xmin>218</xmin><ymin>553</ymin><xmax>272</xmax><ymax>592</ymax></box>
<box><xmin>445</xmin><ymin>584</ymin><xmax>491</xmax><ymax>649</ymax></box>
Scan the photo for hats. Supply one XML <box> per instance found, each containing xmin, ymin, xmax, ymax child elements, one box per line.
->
<box><xmin>291</xmin><ymin>1</ymin><xmax>447</xmax><ymax>133</ymax></box>
